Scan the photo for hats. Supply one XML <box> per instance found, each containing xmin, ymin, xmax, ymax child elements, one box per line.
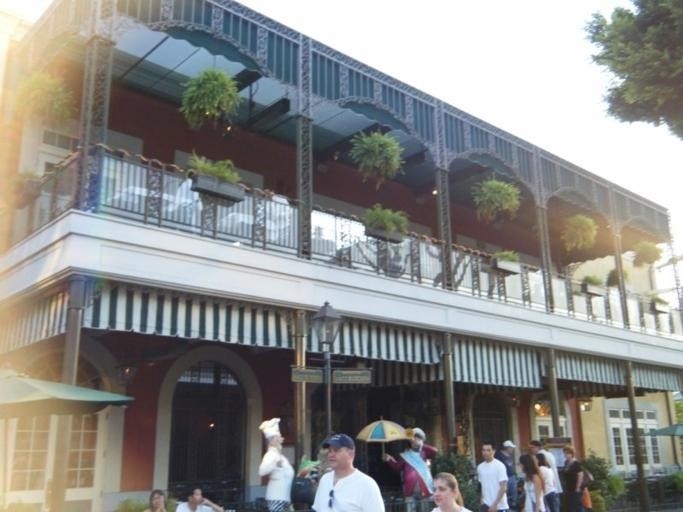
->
<box><xmin>321</xmin><ymin>433</ymin><xmax>355</xmax><ymax>450</ymax></box>
<box><xmin>413</xmin><ymin>428</ymin><xmax>425</xmax><ymax>440</ymax></box>
<box><xmin>503</xmin><ymin>440</ymin><xmax>516</xmax><ymax>448</ymax></box>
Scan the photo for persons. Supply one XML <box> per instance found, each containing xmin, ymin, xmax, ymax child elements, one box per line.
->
<box><xmin>477</xmin><ymin>441</ymin><xmax>510</xmax><ymax>512</ymax></box>
<box><xmin>258</xmin><ymin>188</ymin><xmax>291</xmax><ymax>246</ymax></box>
<box><xmin>144</xmin><ymin>489</ymin><xmax>167</xmax><ymax>512</ymax></box>
<box><xmin>310</xmin><ymin>434</ymin><xmax>385</xmax><ymax>512</ymax></box>
<box><xmin>495</xmin><ymin>440</ymin><xmax>593</xmax><ymax>512</ymax></box>
<box><xmin>381</xmin><ymin>427</ymin><xmax>437</xmax><ymax>512</ymax></box>
<box><xmin>430</xmin><ymin>472</ymin><xmax>473</xmax><ymax>512</ymax></box>
<box><xmin>175</xmin><ymin>488</ymin><xmax>225</xmax><ymax>512</ymax></box>
<box><xmin>258</xmin><ymin>417</ymin><xmax>295</xmax><ymax>512</ymax></box>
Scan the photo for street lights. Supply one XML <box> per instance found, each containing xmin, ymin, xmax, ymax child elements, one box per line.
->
<box><xmin>312</xmin><ymin>303</ymin><xmax>343</xmax><ymax>441</ymax></box>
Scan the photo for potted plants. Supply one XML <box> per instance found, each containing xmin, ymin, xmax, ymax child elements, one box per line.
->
<box><xmin>363</xmin><ymin>202</ymin><xmax>410</xmax><ymax>241</ymax></box>
<box><xmin>177</xmin><ymin>68</ymin><xmax>247</xmax><ymax>132</ymax></box>
<box><xmin>648</xmin><ymin>296</ymin><xmax>670</xmax><ymax>313</ymax></box>
<box><xmin>633</xmin><ymin>241</ymin><xmax>662</xmax><ymax>267</ymax></box>
<box><xmin>350</xmin><ymin>127</ymin><xmax>405</xmax><ymax>182</ymax></box>
<box><xmin>183</xmin><ymin>150</ymin><xmax>246</xmax><ymax>203</ymax></box>
<box><xmin>490</xmin><ymin>248</ymin><xmax>521</xmax><ymax>275</ymax></box>
<box><xmin>580</xmin><ymin>275</ymin><xmax>606</xmax><ymax>297</ymax></box>
<box><xmin>470</xmin><ymin>180</ymin><xmax>521</xmax><ymax>226</ymax></box>
<box><xmin>559</xmin><ymin>213</ymin><xmax>601</xmax><ymax>254</ymax></box>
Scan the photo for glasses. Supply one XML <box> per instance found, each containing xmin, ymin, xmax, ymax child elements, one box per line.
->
<box><xmin>328</xmin><ymin>490</ymin><xmax>334</xmax><ymax>508</ymax></box>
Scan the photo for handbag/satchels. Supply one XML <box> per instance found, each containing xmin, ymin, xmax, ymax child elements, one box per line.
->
<box><xmin>582</xmin><ymin>467</ymin><xmax>594</xmax><ymax>489</ymax></box>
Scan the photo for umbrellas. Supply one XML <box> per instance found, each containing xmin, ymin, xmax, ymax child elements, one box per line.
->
<box><xmin>356</xmin><ymin>416</ymin><xmax>407</xmax><ymax>462</ymax></box>
<box><xmin>0</xmin><ymin>363</ymin><xmax>136</xmax><ymax>512</ymax></box>
<box><xmin>644</xmin><ymin>423</ymin><xmax>683</xmax><ymax>438</ymax></box>
